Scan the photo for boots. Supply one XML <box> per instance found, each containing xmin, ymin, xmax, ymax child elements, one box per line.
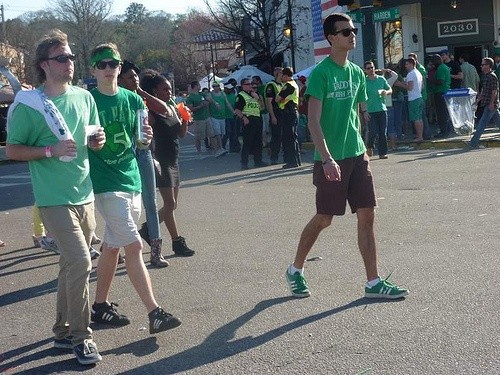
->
<box><xmin>100</xmin><ymin>242</ymin><xmax>125</xmax><ymax>264</ymax></box>
<box><xmin>172</xmin><ymin>236</ymin><xmax>196</xmax><ymax>255</ymax></box>
<box><xmin>138</xmin><ymin>221</ymin><xmax>151</xmax><ymax>247</ymax></box>
<box><xmin>150</xmin><ymin>239</ymin><xmax>169</xmax><ymax>267</ymax></box>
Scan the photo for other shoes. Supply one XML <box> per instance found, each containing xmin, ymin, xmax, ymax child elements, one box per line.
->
<box><xmin>362</xmin><ymin>118</ymin><xmax>477</xmax><ymax>159</ymax></box>
<box><xmin>196</xmin><ymin>141</ymin><xmax>303</xmax><ymax>168</ymax></box>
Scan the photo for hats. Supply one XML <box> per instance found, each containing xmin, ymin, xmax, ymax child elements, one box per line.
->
<box><xmin>296</xmin><ymin>75</ymin><xmax>306</xmax><ymax>84</ymax></box>
<box><xmin>212</xmin><ymin>81</ymin><xmax>220</xmax><ymax>88</ymax></box>
<box><xmin>224</xmin><ymin>84</ymin><xmax>235</xmax><ymax>89</ymax></box>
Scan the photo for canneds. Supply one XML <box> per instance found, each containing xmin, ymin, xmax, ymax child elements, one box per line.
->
<box><xmin>136</xmin><ymin>109</ymin><xmax>148</xmax><ymax>143</ymax></box>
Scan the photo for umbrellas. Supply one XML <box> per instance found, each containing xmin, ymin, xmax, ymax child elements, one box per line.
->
<box><xmin>218</xmin><ymin>66</ymin><xmax>276</xmax><ymax>85</ymax></box>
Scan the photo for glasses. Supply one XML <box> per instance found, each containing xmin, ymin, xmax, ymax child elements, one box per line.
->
<box><xmin>44</xmin><ymin>53</ymin><xmax>77</xmax><ymax>63</ymax></box>
<box><xmin>333</xmin><ymin>27</ymin><xmax>359</xmax><ymax>37</ymax></box>
<box><xmin>252</xmin><ymin>87</ymin><xmax>259</xmax><ymax>90</ymax></box>
<box><xmin>243</xmin><ymin>83</ymin><xmax>251</xmax><ymax>86</ymax></box>
<box><xmin>94</xmin><ymin>59</ymin><xmax>120</xmax><ymax>70</ymax></box>
<box><xmin>480</xmin><ymin>63</ymin><xmax>488</xmax><ymax>67</ymax></box>
<box><xmin>366</xmin><ymin>66</ymin><xmax>374</xmax><ymax>71</ymax></box>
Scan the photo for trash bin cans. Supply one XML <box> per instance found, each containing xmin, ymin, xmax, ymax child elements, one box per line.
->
<box><xmin>442</xmin><ymin>88</ymin><xmax>478</xmax><ymax>136</ymax></box>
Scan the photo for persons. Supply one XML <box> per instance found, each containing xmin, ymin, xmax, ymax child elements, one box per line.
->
<box><xmin>5</xmin><ymin>28</ymin><xmax>106</xmax><ymax>365</ymax></box>
<box><xmin>285</xmin><ymin>14</ymin><xmax>410</xmax><ymax>299</ymax></box>
<box><xmin>32</xmin><ymin>60</ymin><xmax>195</xmax><ymax>266</ymax></box>
<box><xmin>88</xmin><ymin>42</ymin><xmax>182</xmax><ymax>334</ymax></box>
<box><xmin>174</xmin><ymin>49</ymin><xmax>500</xmax><ymax>170</ymax></box>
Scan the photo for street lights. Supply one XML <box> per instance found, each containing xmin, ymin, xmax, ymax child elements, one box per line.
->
<box><xmin>286</xmin><ymin>0</ymin><xmax>295</xmax><ymax>76</ymax></box>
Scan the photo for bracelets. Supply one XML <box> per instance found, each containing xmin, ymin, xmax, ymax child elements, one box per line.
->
<box><xmin>322</xmin><ymin>158</ymin><xmax>334</xmax><ymax>165</ymax></box>
<box><xmin>44</xmin><ymin>146</ymin><xmax>52</xmax><ymax>158</ymax></box>
<box><xmin>241</xmin><ymin>115</ymin><xmax>245</xmax><ymax>119</ymax></box>
<box><xmin>215</xmin><ymin>102</ymin><xmax>218</xmax><ymax>105</ymax></box>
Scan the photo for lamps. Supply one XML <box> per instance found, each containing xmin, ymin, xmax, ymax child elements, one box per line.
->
<box><xmin>450</xmin><ymin>0</ymin><xmax>458</xmax><ymax>8</ymax></box>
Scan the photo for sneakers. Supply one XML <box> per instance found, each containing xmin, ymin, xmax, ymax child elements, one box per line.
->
<box><xmin>41</xmin><ymin>236</ymin><xmax>61</xmax><ymax>254</ymax></box>
<box><xmin>53</xmin><ymin>335</ymin><xmax>74</xmax><ymax>349</ymax></box>
<box><xmin>91</xmin><ymin>302</ymin><xmax>130</xmax><ymax>326</ymax></box>
<box><xmin>32</xmin><ymin>234</ymin><xmax>46</xmax><ymax>248</ymax></box>
<box><xmin>72</xmin><ymin>339</ymin><xmax>101</xmax><ymax>364</ymax></box>
<box><xmin>88</xmin><ymin>247</ymin><xmax>100</xmax><ymax>260</ymax></box>
<box><xmin>148</xmin><ymin>307</ymin><xmax>182</xmax><ymax>334</ymax></box>
<box><xmin>284</xmin><ymin>268</ymin><xmax>311</xmax><ymax>298</ymax></box>
<box><xmin>91</xmin><ymin>235</ymin><xmax>101</xmax><ymax>245</ymax></box>
<box><xmin>365</xmin><ymin>271</ymin><xmax>409</xmax><ymax>299</ymax></box>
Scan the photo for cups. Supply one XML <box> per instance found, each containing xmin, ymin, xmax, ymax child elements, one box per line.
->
<box><xmin>176</xmin><ymin>102</ymin><xmax>191</xmax><ymax>120</ymax></box>
<box><xmin>378</xmin><ymin>90</ymin><xmax>384</xmax><ymax>99</ymax></box>
<box><xmin>84</xmin><ymin>125</ymin><xmax>101</xmax><ymax>147</ymax></box>
